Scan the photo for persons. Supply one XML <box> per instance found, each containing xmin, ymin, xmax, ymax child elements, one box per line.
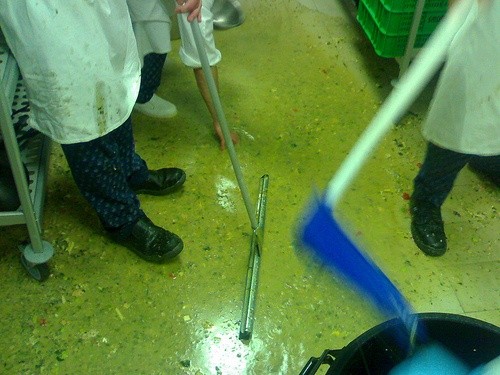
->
<box><xmin>408</xmin><ymin>0</ymin><xmax>500</xmax><ymax>257</ymax></box>
<box><xmin>0</xmin><ymin>0</ymin><xmax>240</xmax><ymax>263</ymax></box>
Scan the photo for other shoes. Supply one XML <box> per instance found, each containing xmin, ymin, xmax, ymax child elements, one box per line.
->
<box><xmin>132</xmin><ymin>95</ymin><xmax>177</xmax><ymax>118</ymax></box>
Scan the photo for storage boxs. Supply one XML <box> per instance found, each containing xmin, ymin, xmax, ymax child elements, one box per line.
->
<box><xmin>356</xmin><ymin>0</ymin><xmax>449</xmax><ymax>58</ymax></box>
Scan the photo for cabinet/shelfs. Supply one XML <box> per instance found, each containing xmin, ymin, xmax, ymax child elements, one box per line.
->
<box><xmin>0</xmin><ymin>31</ymin><xmax>53</xmax><ymax>281</ymax></box>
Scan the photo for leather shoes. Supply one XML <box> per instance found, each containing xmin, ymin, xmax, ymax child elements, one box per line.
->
<box><xmin>137</xmin><ymin>166</ymin><xmax>187</xmax><ymax>192</ymax></box>
<box><xmin>111</xmin><ymin>212</ymin><xmax>183</xmax><ymax>262</ymax></box>
<box><xmin>408</xmin><ymin>196</ymin><xmax>449</xmax><ymax>256</ymax></box>
